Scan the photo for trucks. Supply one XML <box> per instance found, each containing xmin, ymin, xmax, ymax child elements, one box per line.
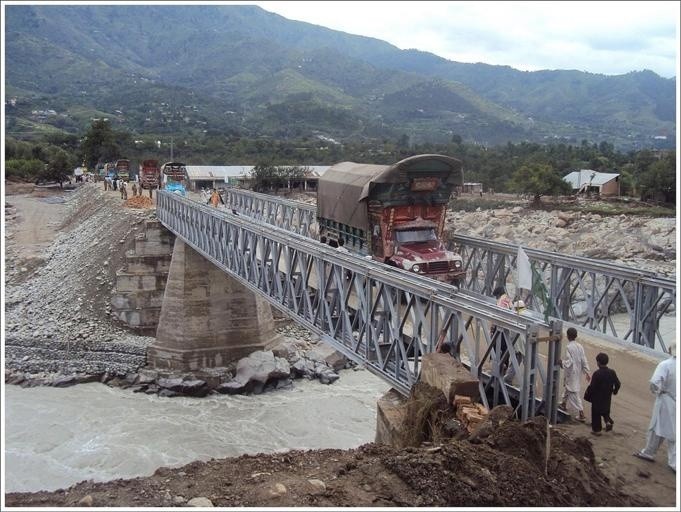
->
<box><xmin>139</xmin><ymin>159</ymin><xmax>160</xmax><ymax>190</ymax></box>
<box><xmin>94</xmin><ymin>160</ymin><xmax>131</xmax><ymax>184</ymax></box>
<box><xmin>160</xmin><ymin>162</ymin><xmax>186</xmax><ymax>198</ymax></box>
<box><xmin>316</xmin><ymin>154</ymin><xmax>466</xmax><ymax>301</ymax></box>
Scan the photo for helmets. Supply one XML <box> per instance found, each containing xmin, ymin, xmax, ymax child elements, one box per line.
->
<box><xmin>512</xmin><ymin>300</ymin><xmax>525</xmax><ymax>309</ymax></box>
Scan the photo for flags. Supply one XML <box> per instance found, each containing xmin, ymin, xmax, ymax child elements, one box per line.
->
<box><xmin>517</xmin><ymin>246</ymin><xmax>532</xmax><ymax>291</ymax></box>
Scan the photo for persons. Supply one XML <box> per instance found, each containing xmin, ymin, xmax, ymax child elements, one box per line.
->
<box><xmin>490</xmin><ymin>286</ymin><xmax>513</xmax><ymax>370</ymax></box>
<box><xmin>558</xmin><ymin>327</ymin><xmax>592</xmax><ymax>420</ymax></box>
<box><xmin>335</xmin><ymin>238</ymin><xmax>349</xmax><ymax>252</ymax></box>
<box><xmin>211</xmin><ymin>188</ymin><xmax>219</xmax><ymax>208</ymax></box>
<box><xmin>502</xmin><ymin>300</ymin><xmax>526</xmax><ymax>384</ymax></box>
<box><xmin>635</xmin><ymin>342</ymin><xmax>676</xmax><ymax>472</ymax></box>
<box><xmin>584</xmin><ymin>353</ymin><xmax>621</xmax><ymax>435</ymax></box>
<box><xmin>104</xmin><ymin>176</ymin><xmax>142</xmax><ymax>201</ymax></box>
<box><xmin>149</xmin><ymin>184</ymin><xmax>152</xmax><ymax>199</ymax></box>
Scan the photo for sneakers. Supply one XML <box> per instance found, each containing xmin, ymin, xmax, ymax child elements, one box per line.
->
<box><xmin>574</xmin><ymin>415</ymin><xmax>587</xmax><ymax>422</ymax></box>
<box><xmin>590</xmin><ymin>430</ymin><xmax>603</xmax><ymax>436</ymax></box>
<box><xmin>605</xmin><ymin>421</ymin><xmax>613</xmax><ymax>432</ymax></box>
<box><xmin>557</xmin><ymin>403</ymin><xmax>566</xmax><ymax>411</ymax></box>
